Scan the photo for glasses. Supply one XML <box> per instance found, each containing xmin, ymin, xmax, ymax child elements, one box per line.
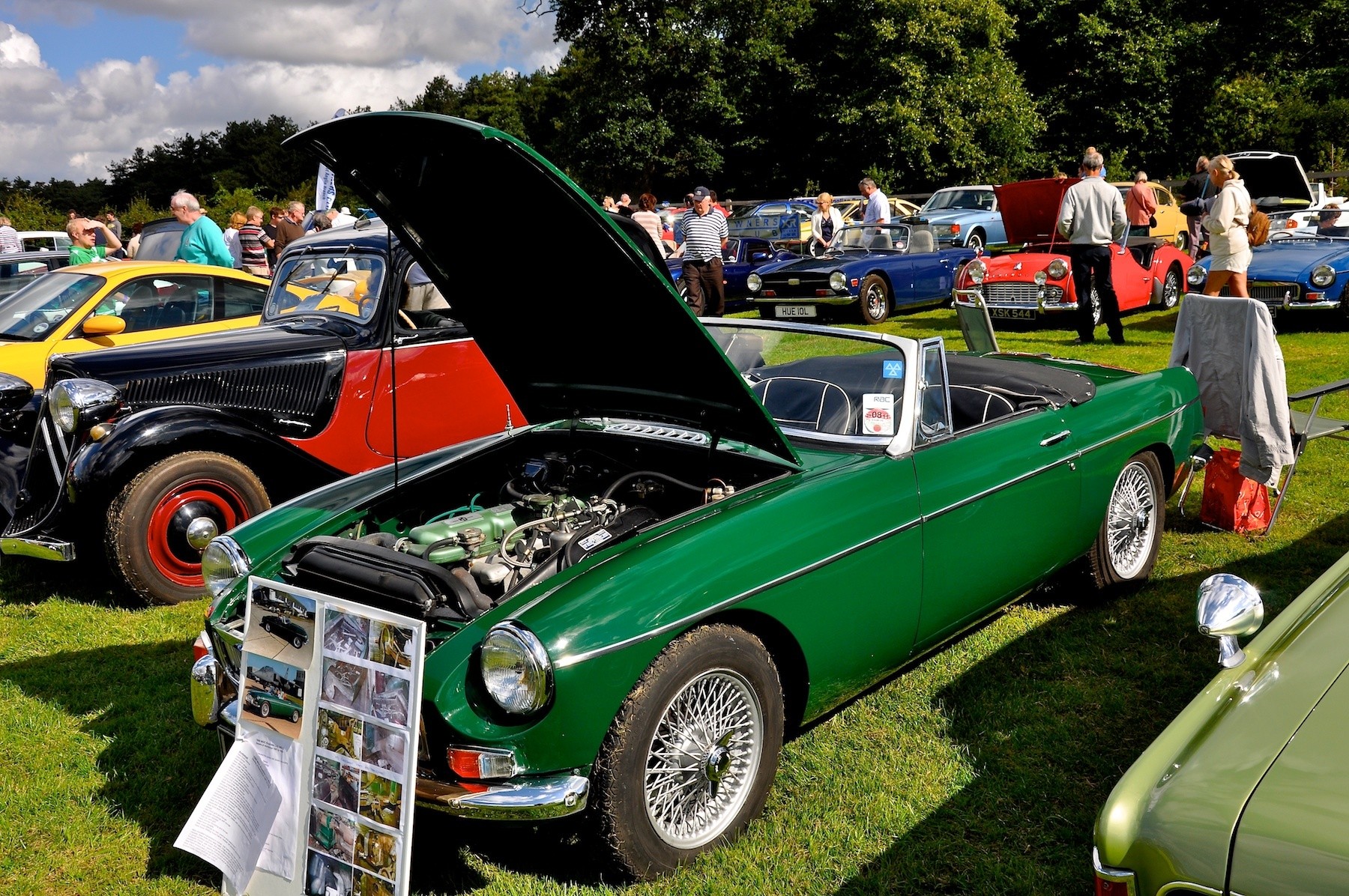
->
<box><xmin>818</xmin><ymin>202</ymin><xmax>825</xmax><ymax>204</ymax></box>
<box><xmin>861</xmin><ymin>181</ymin><xmax>869</xmax><ymax>186</ymax></box>
<box><xmin>275</xmin><ymin>215</ymin><xmax>282</xmax><ymax>219</ymax></box>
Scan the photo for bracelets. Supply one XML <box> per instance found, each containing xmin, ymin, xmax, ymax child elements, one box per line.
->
<box><xmin>122</xmin><ymin>295</ymin><xmax>128</xmax><ymax>303</ymax></box>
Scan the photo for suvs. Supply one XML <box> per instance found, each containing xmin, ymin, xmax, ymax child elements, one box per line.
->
<box><xmin>0</xmin><ymin>216</ymin><xmax>768</xmax><ymax>609</ymax></box>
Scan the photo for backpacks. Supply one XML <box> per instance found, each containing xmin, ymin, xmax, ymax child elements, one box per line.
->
<box><xmin>1234</xmin><ymin>193</ymin><xmax>1270</xmax><ymax>247</ymax></box>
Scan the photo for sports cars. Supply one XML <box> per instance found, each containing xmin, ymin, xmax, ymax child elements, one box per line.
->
<box><xmin>664</xmin><ymin>236</ymin><xmax>803</xmax><ymax>317</ymax></box>
<box><xmin>951</xmin><ymin>177</ymin><xmax>1195</xmax><ymax>330</ymax></box>
<box><xmin>188</xmin><ymin>110</ymin><xmax>1214</xmax><ymax>886</ymax></box>
<box><xmin>0</xmin><ymin>204</ymin><xmax>371</xmax><ymax>394</ymax></box>
<box><xmin>1188</xmin><ymin>208</ymin><xmax>1349</xmax><ymax>330</ymax></box>
<box><xmin>745</xmin><ymin>216</ymin><xmax>990</xmax><ymax>325</ymax></box>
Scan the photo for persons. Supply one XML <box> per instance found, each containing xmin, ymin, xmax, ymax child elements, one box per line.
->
<box><xmin>169</xmin><ymin>190</ymin><xmax>234</xmax><ymax>319</ymax></box>
<box><xmin>810</xmin><ymin>193</ymin><xmax>844</xmax><ymax>257</ymax></box>
<box><xmin>1056</xmin><ymin>152</ymin><xmax>1127</xmax><ymax>346</ymax></box>
<box><xmin>67</xmin><ymin>209</ymin><xmax>77</xmax><ymax>219</ymax></box>
<box><xmin>1315</xmin><ymin>202</ymin><xmax>1341</xmax><ymax>229</ymax></box>
<box><xmin>126</xmin><ymin>220</ymin><xmax>145</xmax><ymax>259</ymax></box>
<box><xmin>1199</xmin><ymin>153</ymin><xmax>1254</xmax><ymax>299</ymax></box>
<box><xmin>599</xmin><ymin>191</ymin><xmax>667</xmax><ymax>259</ymax></box>
<box><xmin>1124</xmin><ymin>170</ymin><xmax>1156</xmax><ymax>237</ymax></box>
<box><xmin>0</xmin><ymin>216</ymin><xmax>23</xmax><ymax>275</ymax></box>
<box><xmin>1181</xmin><ymin>155</ymin><xmax>1222</xmax><ymax>260</ymax></box>
<box><xmin>680</xmin><ymin>186</ymin><xmax>731</xmax><ymax>317</ymax></box>
<box><xmin>105</xmin><ymin>211</ymin><xmax>123</xmax><ymax>258</ymax></box>
<box><xmin>404</xmin><ymin>262</ymin><xmax>450</xmax><ymax>311</ymax></box>
<box><xmin>365</xmin><ymin>269</ymin><xmax>457</xmax><ymax>328</ymax></box>
<box><xmin>857</xmin><ymin>178</ymin><xmax>891</xmax><ymax>248</ymax></box>
<box><xmin>1079</xmin><ymin>147</ymin><xmax>1107</xmax><ymax>181</ymax></box>
<box><xmin>94</xmin><ymin>218</ymin><xmax>107</xmax><ymax>244</ymax></box>
<box><xmin>224</xmin><ymin>201</ymin><xmax>359</xmax><ymax>279</ymax></box>
<box><xmin>66</xmin><ymin>217</ymin><xmax>130</xmax><ymax>316</ymax></box>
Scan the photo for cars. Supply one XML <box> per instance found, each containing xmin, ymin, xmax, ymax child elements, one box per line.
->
<box><xmin>1087</xmin><ymin>549</ymin><xmax>1349</xmax><ymax>895</ymax></box>
<box><xmin>0</xmin><ymin>217</ymin><xmax>192</xmax><ymax>307</ymax></box>
<box><xmin>655</xmin><ymin>150</ymin><xmax>1349</xmax><ymax>270</ymax></box>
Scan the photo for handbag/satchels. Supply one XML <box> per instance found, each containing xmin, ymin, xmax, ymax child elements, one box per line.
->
<box><xmin>1150</xmin><ymin>216</ymin><xmax>1157</xmax><ymax>228</ymax></box>
<box><xmin>1198</xmin><ymin>447</ymin><xmax>1272</xmax><ymax>533</ymax></box>
<box><xmin>1180</xmin><ymin>197</ymin><xmax>1206</xmax><ymax>216</ymax></box>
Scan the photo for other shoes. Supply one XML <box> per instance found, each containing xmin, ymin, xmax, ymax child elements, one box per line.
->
<box><xmin>1075</xmin><ymin>338</ymin><xmax>1082</xmax><ymax>343</ymax></box>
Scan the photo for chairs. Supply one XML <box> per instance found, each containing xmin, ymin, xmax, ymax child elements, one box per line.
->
<box><xmin>908</xmin><ymin>230</ymin><xmax>934</xmax><ymax>254</ymax></box>
<box><xmin>871</xmin><ymin>233</ymin><xmax>894</xmax><ymax>249</ymax></box>
<box><xmin>751</xmin><ymin>377</ymin><xmax>858</xmax><ymax>437</ymax></box>
<box><xmin>859</xmin><ymin>382</ymin><xmax>1015</xmax><ymax>435</ymax></box>
<box><xmin>122</xmin><ymin>285</ymin><xmax>155</xmax><ymax>331</ymax></box>
<box><xmin>153</xmin><ymin>286</ymin><xmax>198</xmax><ymax>326</ymax></box>
<box><xmin>1172</xmin><ymin>295</ymin><xmax>1349</xmax><ymax>536</ymax></box>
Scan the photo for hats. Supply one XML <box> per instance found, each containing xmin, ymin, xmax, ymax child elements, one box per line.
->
<box><xmin>692</xmin><ymin>186</ymin><xmax>710</xmax><ymax>202</ymax></box>
<box><xmin>199</xmin><ymin>208</ymin><xmax>208</xmax><ymax>213</ymax></box>
<box><xmin>340</xmin><ymin>207</ymin><xmax>351</xmax><ymax>215</ymax></box>
